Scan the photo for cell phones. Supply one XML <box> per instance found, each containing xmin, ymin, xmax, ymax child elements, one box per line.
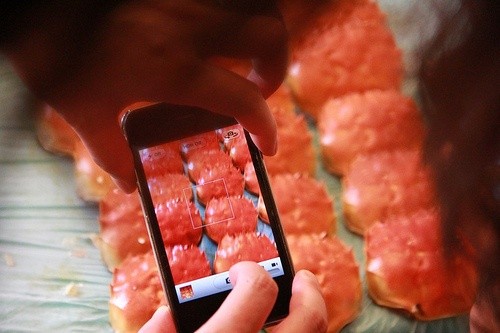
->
<box><xmin>122</xmin><ymin>103</ymin><xmax>299</xmax><ymax>333</ymax></box>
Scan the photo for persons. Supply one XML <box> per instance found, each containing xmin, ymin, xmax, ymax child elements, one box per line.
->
<box><xmin>1</xmin><ymin>0</ymin><xmax>500</xmax><ymax>333</ymax></box>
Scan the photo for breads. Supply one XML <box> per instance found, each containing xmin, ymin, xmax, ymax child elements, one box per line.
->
<box><xmin>36</xmin><ymin>0</ymin><xmax>472</xmax><ymax>331</ymax></box>
